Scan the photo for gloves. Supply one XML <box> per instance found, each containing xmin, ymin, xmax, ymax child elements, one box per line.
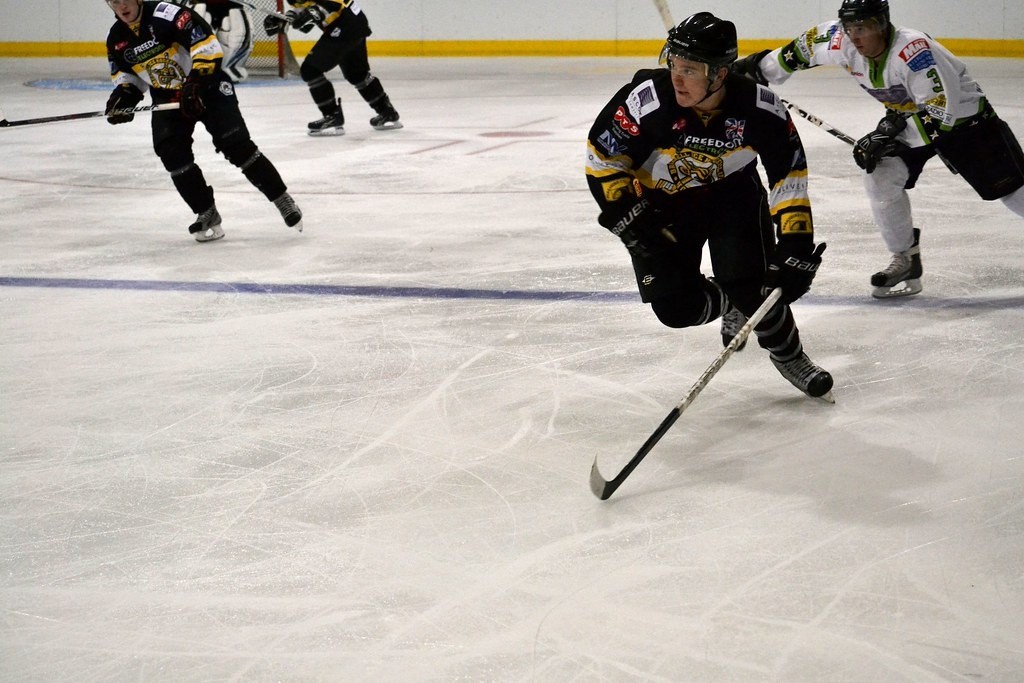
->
<box><xmin>292</xmin><ymin>4</ymin><xmax>326</xmax><ymax>33</ymax></box>
<box><xmin>732</xmin><ymin>49</ymin><xmax>773</xmax><ymax>86</ymax></box>
<box><xmin>104</xmin><ymin>82</ymin><xmax>144</xmax><ymax>125</ymax></box>
<box><xmin>759</xmin><ymin>238</ymin><xmax>826</xmax><ymax>306</ymax></box>
<box><xmin>598</xmin><ymin>189</ymin><xmax>676</xmax><ymax>256</ymax></box>
<box><xmin>853</xmin><ymin>108</ymin><xmax>908</xmax><ymax>174</ymax></box>
<box><xmin>179</xmin><ymin>68</ymin><xmax>214</xmax><ymax>120</ymax></box>
<box><xmin>263</xmin><ymin>9</ymin><xmax>297</xmax><ymax>36</ymax></box>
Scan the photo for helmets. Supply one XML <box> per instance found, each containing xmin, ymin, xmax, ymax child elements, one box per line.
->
<box><xmin>667</xmin><ymin>11</ymin><xmax>737</xmax><ymax>82</ymax></box>
<box><xmin>838</xmin><ymin>0</ymin><xmax>891</xmax><ymax>29</ymax></box>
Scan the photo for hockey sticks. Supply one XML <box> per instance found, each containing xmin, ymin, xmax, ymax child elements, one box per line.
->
<box><xmin>653</xmin><ymin>0</ymin><xmax>857</xmax><ymax>148</ymax></box>
<box><xmin>229</xmin><ymin>0</ymin><xmax>295</xmax><ymax>24</ymax></box>
<box><xmin>587</xmin><ymin>239</ymin><xmax>828</xmax><ymax>503</ymax></box>
<box><xmin>0</xmin><ymin>103</ymin><xmax>180</xmax><ymax>129</ymax></box>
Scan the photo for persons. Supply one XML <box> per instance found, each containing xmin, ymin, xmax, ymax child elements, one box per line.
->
<box><xmin>586</xmin><ymin>12</ymin><xmax>836</xmax><ymax>405</ymax></box>
<box><xmin>103</xmin><ymin>0</ymin><xmax>306</xmax><ymax>242</ymax></box>
<box><xmin>735</xmin><ymin>0</ymin><xmax>1024</xmax><ymax>299</ymax></box>
<box><xmin>264</xmin><ymin>0</ymin><xmax>403</xmax><ymax>138</ymax></box>
<box><xmin>167</xmin><ymin>0</ymin><xmax>254</xmax><ymax>85</ymax></box>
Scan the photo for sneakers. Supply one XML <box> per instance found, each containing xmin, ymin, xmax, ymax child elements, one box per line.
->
<box><xmin>274</xmin><ymin>191</ymin><xmax>303</xmax><ymax>232</ymax></box>
<box><xmin>369</xmin><ymin>95</ymin><xmax>404</xmax><ymax>130</ymax></box>
<box><xmin>708</xmin><ymin>275</ymin><xmax>749</xmax><ymax>352</ymax></box>
<box><xmin>308</xmin><ymin>96</ymin><xmax>346</xmax><ymax>136</ymax></box>
<box><xmin>871</xmin><ymin>227</ymin><xmax>923</xmax><ymax>299</ymax></box>
<box><xmin>769</xmin><ymin>350</ymin><xmax>835</xmax><ymax>404</ymax></box>
<box><xmin>189</xmin><ymin>185</ymin><xmax>225</xmax><ymax>243</ymax></box>
